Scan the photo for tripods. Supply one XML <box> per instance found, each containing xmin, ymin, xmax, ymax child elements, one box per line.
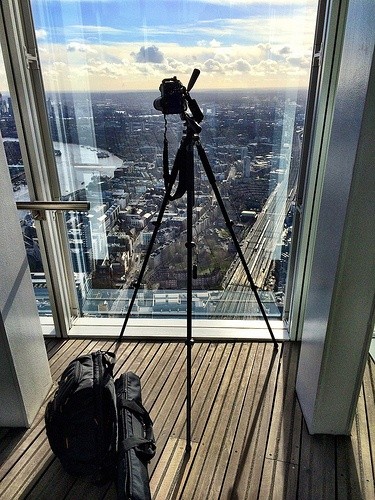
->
<box><xmin>109</xmin><ymin>118</ymin><xmax>279</xmax><ymax>455</ymax></box>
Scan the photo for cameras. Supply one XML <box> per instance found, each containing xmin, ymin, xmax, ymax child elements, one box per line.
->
<box><xmin>152</xmin><ymin>76</ymin><xmax>187</xmax><ymax>114</ymax></box>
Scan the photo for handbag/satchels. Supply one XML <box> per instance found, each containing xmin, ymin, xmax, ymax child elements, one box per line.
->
<box><xmin>45</xmin><ymin>350</ymin><xmax>157</xmax><ymax>500</ymax></box>
